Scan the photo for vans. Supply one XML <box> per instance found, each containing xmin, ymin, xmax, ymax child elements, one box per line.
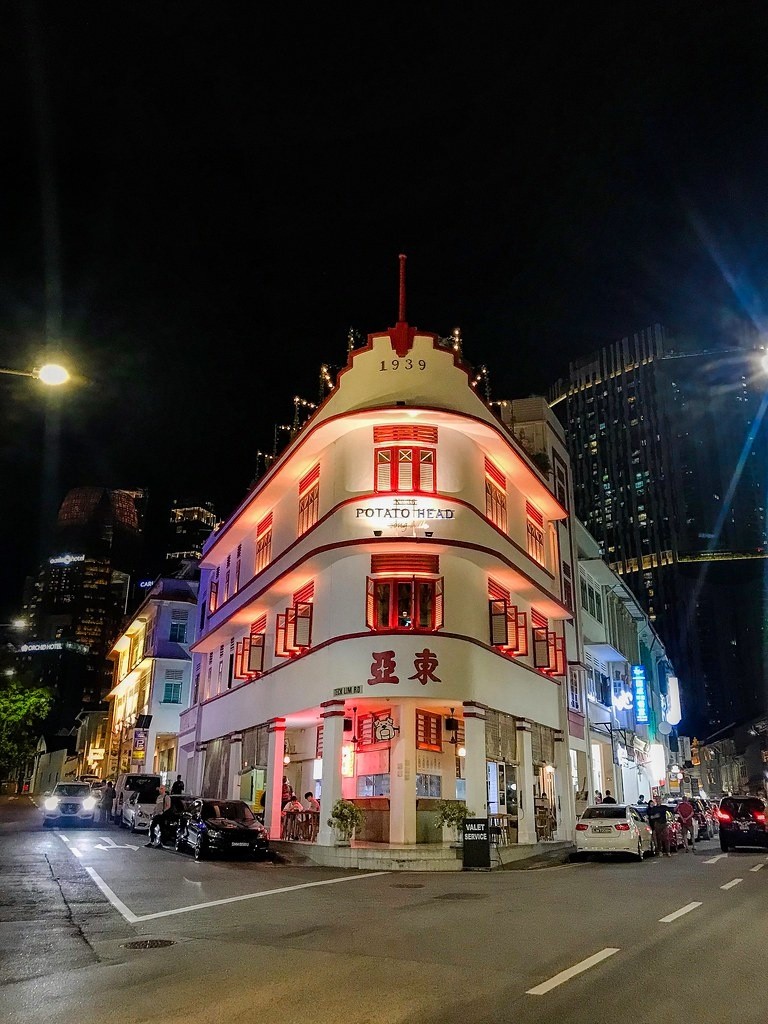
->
<box><xmin>110</xmin><ymin>773</ymin><xmax>162</xmax><ymax>825</ymax></box>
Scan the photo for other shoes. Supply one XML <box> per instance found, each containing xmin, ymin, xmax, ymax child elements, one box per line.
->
<box><xmin>155</xmin><ymin>844</ymin><xmax>163</xmax><ymax>850</ymax></box>
<box><xmin>144</xmin><ymin>843</ymin><xmax>155</xmax><ymax>848</ymax></box>
<box><xmin>658</xmin><ymin>851</ymin><xmax>663</xmax><ymax>858</ymax></box>
<box><xmin>668</xmin><ymin>852</ymin><xmax>671</xmax><ymax>857</ymax></box>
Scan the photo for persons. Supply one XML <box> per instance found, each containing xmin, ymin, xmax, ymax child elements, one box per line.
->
<box><xmin>645</xmin><ymin>800</ymin><xmax>660</xmax><ymax>853</ymax></box>
<box><xmin>282</xmin><ymin>776</ymin><xmax>295</xmax><ymax>811</ymax></box>
<box><xmin>144</xmin><ymin>784</ymin><xmax>171</xmax><ymax>849</ymax></box>
<box><xmin>595</xmin><ymin>792</ymin><xmax>602</xmax><ymax>804</ymax></box>
<box><xmin>650</xmin><ymin>796</ymin><xmax>677</xmax><ymax>858</ymax></box>
<box><xmin>676</xmin><ymin>796</ymin><xmax>697</xmax><ymax>853</ymax></box>
<box><xmin>297</xmin><ymin>791</ymin><xmax>319</xmax><ymax>841</ymax></box>
<box><xmin>283</xmin><ymin>796</ymin><xmax>306</xmax><ymax>841</ymax></box>
<box><xmin>637</xmin><ymin>795</ymin><xmax>644</xmax><ymax>805</ymax></box>
<box><xmin>92</xmin><ymin>779</ymin><xmax>116</xmax><ymax>825</ymax></box>
<box><xmin>171</xmin><ymin>774</ymin><xmax>184</xmax><ymax>795</ymax></box>
<box><xmin>603</xmin><ymin>790</ymin><xmax>616</xmax><ymax>804</ymax></box>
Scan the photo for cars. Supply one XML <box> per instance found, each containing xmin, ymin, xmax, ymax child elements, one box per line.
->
<box><xmin>574</xmin><ymin>803</ymin><xmax>656</xmax><ymax>862</ymax></box>
<box><xmin>77</xmin><ymin>775</ymin><xmax>110</xmax><ymax>796</ymax></box>
<box><xmin>174</xmin><ymin>798</ymin><xmax>269</xmax><ymax>861</ymax></box>
<box><xmin>634</xmin><ymin>797</ymin><xmax>720</xmax><ymax>857</ymax></box>
<box><xmin>148</xmin><ymin>794</ymin><xmax>201</xmax><ymax>847</ymax></box>
<box><xmin>119</xmin><ymin>789</ymin><xmax>171</xmax><ymax>834</ymax></box>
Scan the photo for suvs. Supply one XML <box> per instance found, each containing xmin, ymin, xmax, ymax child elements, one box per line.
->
<box><xmin>718</xmin><ymin>795</ymin><xmax>768</xmax><ymax>853</ymax></box>
<box><xmin>42</xmin><ymin>781</ymin><xmax>102</xmax><ymax>828</ymax></box>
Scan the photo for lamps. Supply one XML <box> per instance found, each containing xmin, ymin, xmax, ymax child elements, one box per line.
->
<box><xmin>373</xmin><ymin>530</ymin><xmax>383</xmax><ymax>536</ymax></box>
<box><xmin>397</xmin><ymin>400</ymin><xmax>405</xmax><ymax>405</ymax></box>
<box><xmin>425</xmin><ymin>531</ymin><xmax>434</xmax><ymax>537</ymax></box>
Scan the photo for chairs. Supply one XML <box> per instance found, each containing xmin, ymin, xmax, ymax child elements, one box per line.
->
<box><xmin>488</xmin><ymin>812</ymin><xmax>554</xmax><ymax>845</ymax></box>
<box><xmin>282</xmin><ymin>811</ymin><xmax>319</xmax><ymax>842</ymax></box>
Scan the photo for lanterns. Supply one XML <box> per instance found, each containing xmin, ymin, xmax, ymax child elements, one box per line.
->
<box><xmin>658</xmin><ymin>721</ymin><xmax>672</xmax><ymax>735</ymax></box>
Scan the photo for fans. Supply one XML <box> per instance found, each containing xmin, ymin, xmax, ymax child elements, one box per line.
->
<box><xmin>344</xmin><ymin>706</ymin><xmax>367</xmax><ymax>744</ymax></box>
<box><xmin>440</xmin><ymin>707</ymin><xmax>463</xmax><ymax>744</ymax></box>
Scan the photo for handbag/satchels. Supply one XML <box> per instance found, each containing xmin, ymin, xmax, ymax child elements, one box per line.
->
<box><xmin>163</xmin><ymin>807</ymin><xmax>175</xmax><ymax>821</ymax></box>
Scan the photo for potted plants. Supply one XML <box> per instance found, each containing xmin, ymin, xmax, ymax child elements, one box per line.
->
<box><xmin>433</xmin><ymin>799</ymin><xmax>476</xmax><ymax>847</ymax></box>
<box><xmin>326</xmin><ymin>798</ymin><xmax>369</xmax><ymax>847</ymax></box>
